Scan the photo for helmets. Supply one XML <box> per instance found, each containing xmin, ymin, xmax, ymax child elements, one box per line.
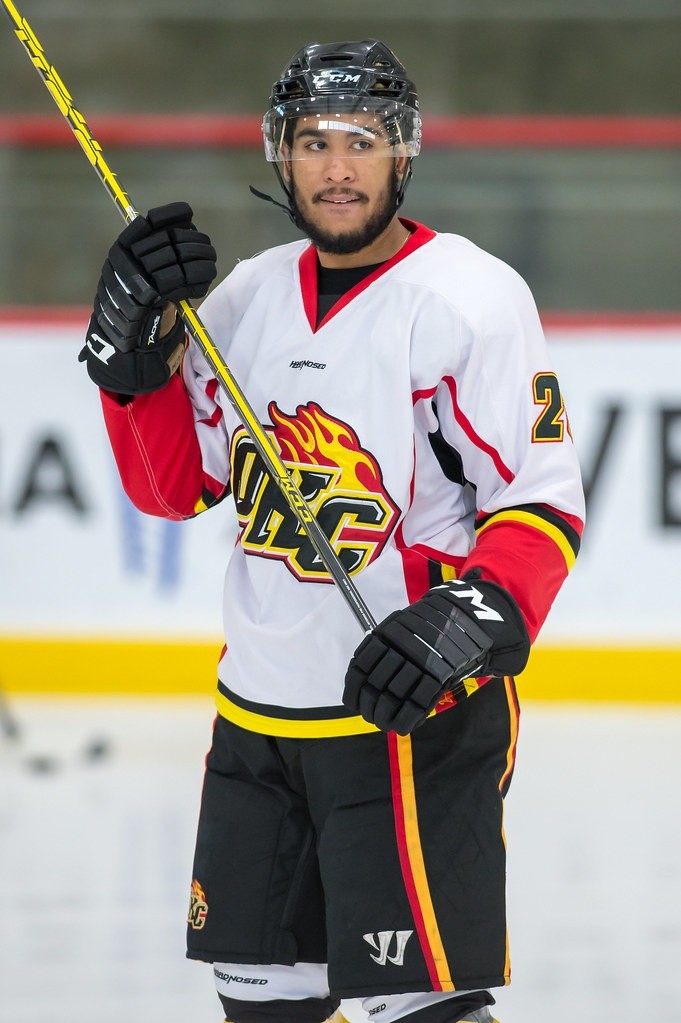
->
<box><xmin>261</xmin><ymin>40</ymin><xmax>422</xmax><ymax>162</ymax></box>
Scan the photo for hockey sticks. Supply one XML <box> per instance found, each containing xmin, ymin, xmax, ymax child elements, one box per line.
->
<box><xmin>0</xmin><ymin>0</ymin><xmax>382</xmax><ymax>638</ymax></box>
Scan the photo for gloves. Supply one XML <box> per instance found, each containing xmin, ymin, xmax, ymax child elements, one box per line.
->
<box><xmin>342</xmin><ymin>566</ymin><xmax>530</xmax><ymax>735</ymax></box>
<box><xmin>78</xmin><ymin>202</ymin><xmax>218</xmax><ymax>395</ymax></box>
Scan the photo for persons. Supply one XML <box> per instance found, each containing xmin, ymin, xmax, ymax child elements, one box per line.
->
<box><xmin>76</xmin><ymin>30</ymin><xmax>584</xmax><ymax>1022</ymax></box>
